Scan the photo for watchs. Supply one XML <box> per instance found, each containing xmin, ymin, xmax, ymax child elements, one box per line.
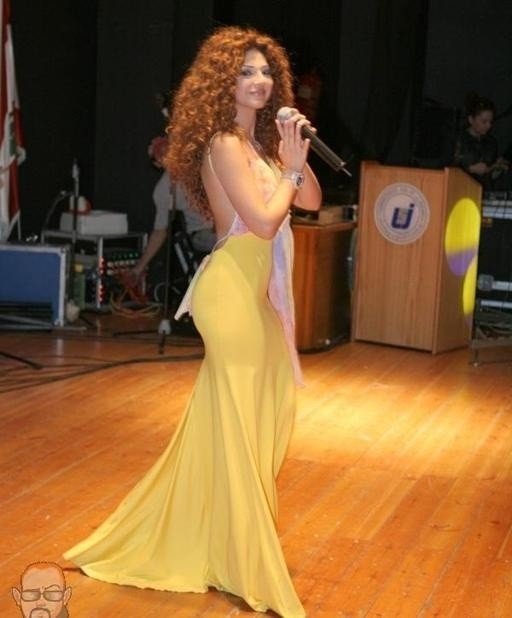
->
<box><xmin>280</xmin><ymin>168</ymin><xmax>306</xmax><ymax>191</ymax></box>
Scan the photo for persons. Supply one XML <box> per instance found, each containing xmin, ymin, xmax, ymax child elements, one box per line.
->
<box><xmin>432</xmin><ymin>93</ymin><xmax>510</xmax><ymax>194</ymax></box>
<box><xmin>63</xmin><ymin>25</ymin><xmax>322</xmax><ymax>618</ymax></box>
<box><xmin>127</xmin><ymin>135</ymin><xmax>217</xmax><ymax>287</ymax></box>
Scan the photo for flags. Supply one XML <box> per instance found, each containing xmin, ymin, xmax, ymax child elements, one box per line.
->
<box><xmin>0</xmin><ymin>0</ymin><xmax>28</xmax><ymax>243</ymax></box>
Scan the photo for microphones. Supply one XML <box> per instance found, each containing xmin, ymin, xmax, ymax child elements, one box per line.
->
<box><xmin>277</xmin><ymin>106</ymin><xmax>352</xmax><ymax>178</ymax></box>
